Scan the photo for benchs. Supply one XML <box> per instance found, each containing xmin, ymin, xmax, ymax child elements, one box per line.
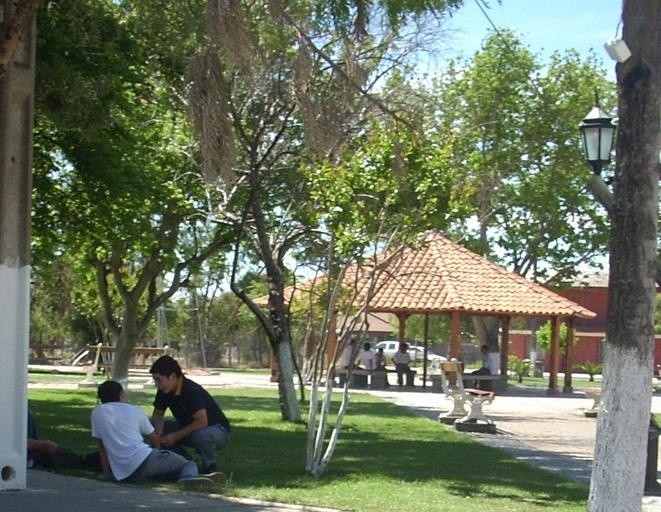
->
<box><xmin>430</xmin><ymin>374</ymin><xmax>508</xmax><ymax>394</ymax></box>
<box><xmin>338</xmin><ymin>368</ymin><xmax>417</xmax><ymax>389</ymax></box>
<box><xmin>435</xmin><ymin>358</ymin><xmax>497</xmax><ymax>434</ymax></box>
<box><xmin>77</xmin><ymin>343</ymin><xmax>169</xmax><ymax>389</ymax></box>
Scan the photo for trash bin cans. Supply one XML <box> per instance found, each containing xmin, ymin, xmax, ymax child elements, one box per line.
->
<box><xmin>430</xmin><ymin>375</ymin><xmax>444</xmax><ymax>393</ymax></box>
<box><xmin>643</xmin><ymin>416</ymin><xmax>661</xmax><ymax>496</ymax></box>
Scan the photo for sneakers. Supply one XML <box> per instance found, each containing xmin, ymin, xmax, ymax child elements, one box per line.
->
<box><xmin>198</xmin><ymin>471</ymin><xmax>226</xmax><ymax>482</ymax></box>
<box><xmin>176</xmin><ymin>477</ymin><xmax>215</xmax><ymax>489</ymax></box>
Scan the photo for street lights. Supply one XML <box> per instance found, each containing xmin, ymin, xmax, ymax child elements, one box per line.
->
<box><xmin>579</xmin><ymin>92</ymin><xmax>616</xmax><ymax>185</ymax></box>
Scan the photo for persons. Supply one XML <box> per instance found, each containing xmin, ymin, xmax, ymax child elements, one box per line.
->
<box><xmin>374</xmin><ymin>347</ymin><xmax>389</xmax><ymax>384</ymax></box>
<box><xmin>391</xmin><ymin>343</ymin><xmax>413</xmax><ymax>387</ymax></box>
<box><xmin>470</xmin><ymin>344</ymin><xmax>500</xmax><ymax>376</ymax></box>
<box><xmin>28</xmin><ymin>412</ymin><xmax>85</xmax><ymax>473</ymax></box>
<box><xmin>343</xmin><ymin>339</ymin><xmax>363</xmax><ymax>370</ymax></box>
<box><xmin>356</xmin><ymin>343</ymin><xmax>378</xmax><ymax>373</ymax></box>
<box><xmin>149</xmin><ymin>356</ymin><xmax>232</xmax><ymax>473</ymax></box>
<box><xmin>90</xmin><ymin>380</ymin><xmax>228</xmax><ymax>489</ymax></box>
